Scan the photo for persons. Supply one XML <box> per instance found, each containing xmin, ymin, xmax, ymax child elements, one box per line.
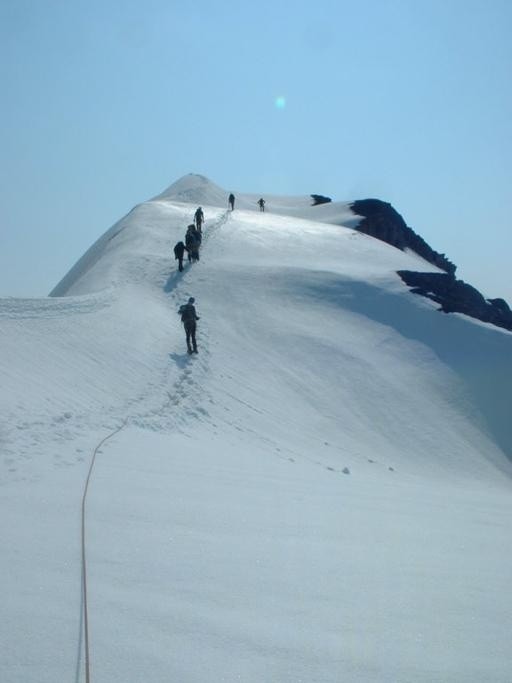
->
<box><xmin>256</xmin><ymin>197</ymin><xmax>266</xmax><ymax>211</ymax></box>
<box><xmin>192</xmin><ymin>207</ymin><xmax>204</xmax><ymax>233</ymax></box>
<box><xmin>177</xmin><ymin>296</ymin><xmax>201</xmax><ymax>354</ymax></box>
<box><xmin>173</xmin><ymin>241</ymin><xmax>192</xmax><ymax>272</ymax></box>
<box><xmin>186</xmin><ymin>224</ymin><xmax>202</xmax><ymax>263</ymax></box>
<box><xmin>228</xmin><ymin>193</ymin><xmax>236</xmax><ymax>210</ymax></box>
